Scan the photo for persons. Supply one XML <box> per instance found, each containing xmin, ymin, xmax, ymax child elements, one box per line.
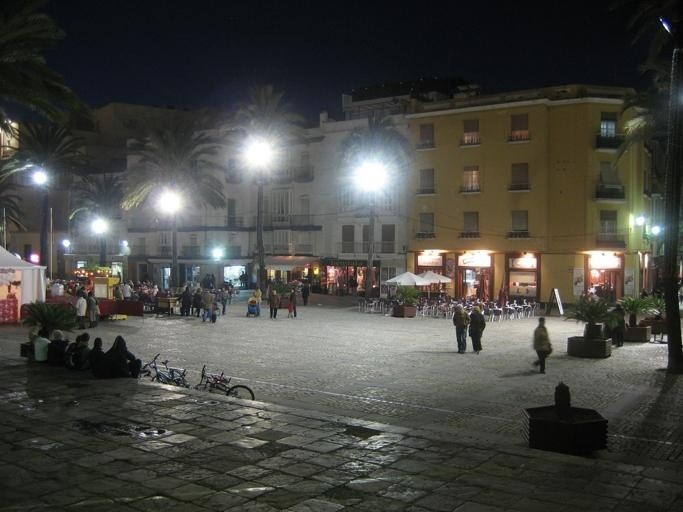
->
<box><xmin>468</xmin><ymin>306</ymin><xmax>486</xmax><ymax>354</ymax></box>
<box><xmin>452</xmin><ymin>304</ymin><xmax>469</xmax><ymax>354</ymax></box>
<box><xmin>45</xmin><ymin>266</ymin><xmax>310</xmax><ymax>330</ymax></box>
<box><xmin>531</xmin><ymin>317</ymin><xmax>552</xmax><ymax>374</ymax></box>
<box><xmin>28</xmin><ymin>326</ymin><xmax>141</xmax><ymax>379</ymax></box>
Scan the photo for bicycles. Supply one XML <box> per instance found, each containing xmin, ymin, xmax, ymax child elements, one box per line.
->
<box><xmin>194</xmin><ymin>364</ymin><xmax>255</xmax><ymax>402</ymax></box>
<box><xmin>141</xmin><ymin>351</ymin><xmax>190</xmax><ymax>388</ymax></box>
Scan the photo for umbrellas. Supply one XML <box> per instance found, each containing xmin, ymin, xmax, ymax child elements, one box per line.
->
<box><xmin>385</xmin><ymin>271</ymin><xmax>430</xmax><ymax>289</ymax></box>
<box><xmin>416</xmin><ymin>270</ymin><xmax>452</xmax><ymax>298</ymax></box>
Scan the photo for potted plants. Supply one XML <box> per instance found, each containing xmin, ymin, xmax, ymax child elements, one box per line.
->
<box><xmin>273</xmin><ymin>280</ymin><xmax>290</xmax><ymax>308</ymax></box>
<box><xmin>563</xmin><ymin>293</ymin><xmax>624</xmax><ymax>341</ymax></box>
<box><xmin>20</xmin><ymin>300</ymin><xmax>78</xmax><ymax>358</ymax></box>
<box><xmin>393</xmin><ymin>286</ymin><xmax>420</xmax><ymax>316</ymax></box>
<box><xmin>614</xmin><ymin>295</ymin><xmax>652</xmax><ymax>342</ymax></box>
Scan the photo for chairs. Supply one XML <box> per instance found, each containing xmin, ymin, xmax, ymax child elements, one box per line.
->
<box><xmin>167</xmin><ymin>286</ymin><xmax>233</xmax><ymax>304</ymax></box>
<box><xmin>357</xmin><ymin>295</ymin><xmax>541</xmax><ymax>323</ymax></box>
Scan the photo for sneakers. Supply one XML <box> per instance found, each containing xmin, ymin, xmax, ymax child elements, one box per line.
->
<box><xmin>79</xmin><ymin>325</ymin><xmax>97</xmax><ymax>329</ymax></box>
<box><xmin>270</xmin><ymin>315</ymin><xmax>277</xmax><ymax>319</ymax></box>
<box><xmin>458</xmin><ymin>348</ymin><xmax>483</xmax><ymax>354</ymax></box>
<box><xmin>294</xmin><ymin>314</ymin><xmax>297</xmax><ymax>317</ymax></box>
<box><xmin>533</xmin><ymin>362</ymin><xmax>546</xmax><ymax>374</ymax></box>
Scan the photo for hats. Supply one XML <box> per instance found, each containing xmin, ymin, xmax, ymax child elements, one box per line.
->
<box><xmin>113</xmin><ymin>335</ymin><xmax>126</xmax><ymax>349</ymax></box>
<box><xmin>53</xmin><ymin>330</ymin><xmax>63</xmax><ymax>339</ymax></box>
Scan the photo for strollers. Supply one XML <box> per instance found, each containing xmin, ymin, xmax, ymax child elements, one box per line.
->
<box><xmin>246</xmin><ymin>297</ymin><xmax>260</xmax><ymax>317</ymax></box>
